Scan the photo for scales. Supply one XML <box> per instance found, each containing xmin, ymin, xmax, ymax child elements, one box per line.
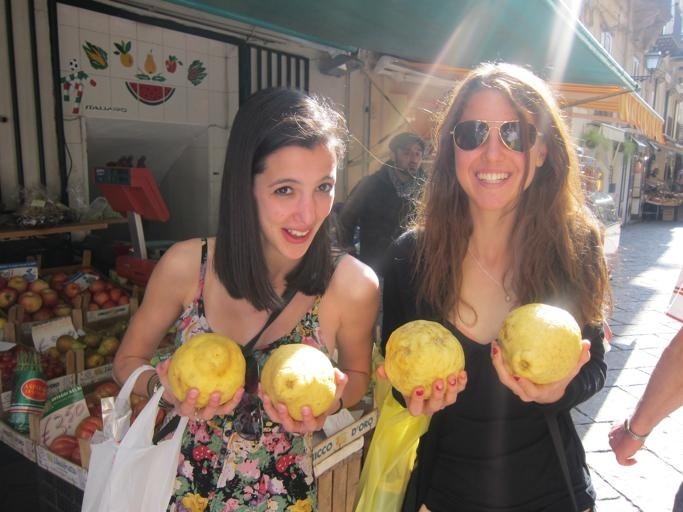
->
<box><xmin>93</xmin><ymin>165</ymin><xmax>171</xmax><ymax>287</ymax></box>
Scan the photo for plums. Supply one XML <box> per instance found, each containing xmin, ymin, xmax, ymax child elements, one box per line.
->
<box><xmin>0</xmin><ymin>344</ymin><xmax>65</xmax><ymax>392</ymax></box>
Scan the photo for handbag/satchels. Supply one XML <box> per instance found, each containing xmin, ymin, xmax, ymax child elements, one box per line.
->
<box><xmin>83</xmin><ymin>364</ymin><xmax>190</xmax><ymax>507</ymax></box>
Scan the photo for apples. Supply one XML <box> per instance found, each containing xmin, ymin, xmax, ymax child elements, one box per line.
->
<box><xmin>166</xmin><ymin>54</ymin><xmax>176</xmax><ymax>74</ymax></box>
<box><xmin>119</xmin><ymin>53</ymin><xmax>133</xmax><ymax>67</ymax></box>
<box><xmin>0</xmin><ymin>265</ymin><xmax>129</xmax><ymax>321</ymax></box>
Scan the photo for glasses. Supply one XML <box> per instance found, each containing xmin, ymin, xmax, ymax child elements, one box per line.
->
<box><xmin>450</xmin><ymin>120</ymin><xmax>542</xmax><ymax>153</ymax></box>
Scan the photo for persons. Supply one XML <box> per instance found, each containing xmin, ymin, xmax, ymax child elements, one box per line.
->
<box><xmin>112</xmin><ymin>84</ymin><xmax>380</xmax><ymax>512</ymax></box>
<box><xmin>608</xmin><ymin>265</ymin><xmax>682</xmax><ymax>511</ymax></box>
<box><xmin>337</xmin><ymin>132</ymin><xmax>428</xmax><ymax>341</ymax></box>
<box><xmin>647</xmin><ymin>167</ymin><xmax>664</xmax><ymax>189</ymax></box>
<box><xmin>365</xmin><ymin>58</ymin><xmax>608</xmax><ymax>512</ymax></box>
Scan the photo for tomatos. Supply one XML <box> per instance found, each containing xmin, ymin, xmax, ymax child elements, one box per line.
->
<box><xmin>51</xmin><ymin>382</ymin><xmax>165</xmax><ymax>466</ymax></box>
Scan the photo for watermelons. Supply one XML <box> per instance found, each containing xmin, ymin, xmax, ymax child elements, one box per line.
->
<box><xmin>124</xmin><ymin>80</ymin><xmax>175</xmax><ymax>105</ymax></box>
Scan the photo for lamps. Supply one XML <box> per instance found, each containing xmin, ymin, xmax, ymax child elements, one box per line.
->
<box><xmin>631</xmin><ymin>46</ymin><xmax>662</xmax><ymax>82</ymax></box>
<box><xmin>321</xmin><ymin>54</ymin><xmax>365</xmax><ymax>77</ymax></box>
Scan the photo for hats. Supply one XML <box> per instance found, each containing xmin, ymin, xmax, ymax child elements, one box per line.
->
<box><xmin>389</xmin><ymin>133</ymin><xmax>425</xmax><ymax>153</ymax></box>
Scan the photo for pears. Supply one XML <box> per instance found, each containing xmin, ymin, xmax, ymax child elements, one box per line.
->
<box><xmin>260</xmin><ymin>343</ymin><xmax>337</xmax><ymax>421</ymax></box>
<box><xmin>384</xmin><ymin>319</ymin><xmax>466</xmax><ymax>399</ymax></box>
<box><xmin>166</xmin><ymin>332</ymin><xmax>247</xmax><ymax>409</ymax></box>
<box><xmin>48</xmin><ymin>333</ymin><xmax>119</xmax><ymax>368</ymax></box>
<box><xmin>144</xmin><ymin>48</ymin><xmax>157</xmax><ymax>75</ymax></box>
<box><xmin>497</xmin><ymin>302</ymin><xmax>583</xmax><ymax>386</ymax></box>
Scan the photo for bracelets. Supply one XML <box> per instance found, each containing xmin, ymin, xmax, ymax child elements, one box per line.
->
<box><xmin>145</xmin><ymin>373</ymin><xmax>170</xmax><ymax>412</ymax></box>
<box><xmin>624</xmin><ymin>417</ymin><xmax>651</xmax><ymax>445</ymax></box>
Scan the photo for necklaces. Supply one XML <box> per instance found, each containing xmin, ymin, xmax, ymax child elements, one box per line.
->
<box><xmin>462</xmin><ymin>236</ymin><xmax>522</xmax><ymax>302</ymax></box>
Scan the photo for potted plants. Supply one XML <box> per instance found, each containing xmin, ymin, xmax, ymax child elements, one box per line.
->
<box><xmin>584</xmin><ymin>127</ymin><xmax>610</xmax><ymax>151</ymax></box>
<box><xmin>615</xmin><ymin>140</ymin><xmax>637</xmax><ymax>162</ymax></box>
